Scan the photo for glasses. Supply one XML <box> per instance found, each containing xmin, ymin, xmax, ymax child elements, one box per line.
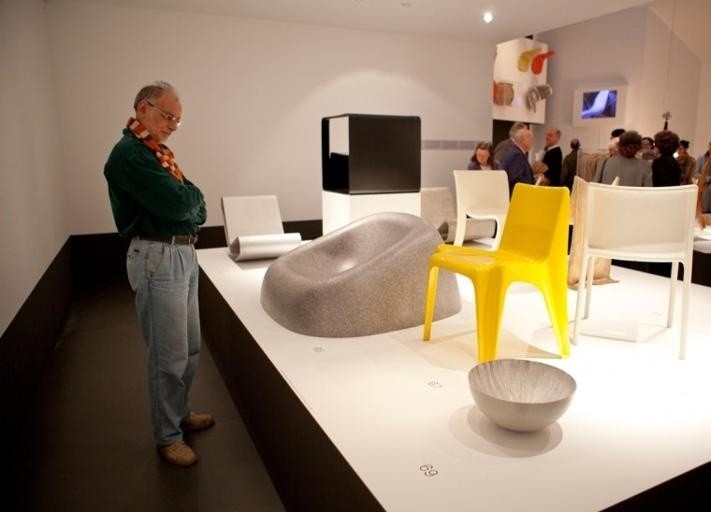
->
<box><xmin>146</xmin><ymin>99</ymin><xmax>183</xmax><ymax>127</ymax></box>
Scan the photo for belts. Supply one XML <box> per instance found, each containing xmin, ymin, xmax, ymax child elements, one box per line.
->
<box><xmin>135</xmin><ymin>234</ymin><xmax>198</xmax><ymax>245</ymax></box>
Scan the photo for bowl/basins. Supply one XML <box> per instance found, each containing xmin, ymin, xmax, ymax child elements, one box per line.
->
<box><xmin>468</xmin><ymin>358</ymin><xmax>576</xmax><ymax>432</ymax></box>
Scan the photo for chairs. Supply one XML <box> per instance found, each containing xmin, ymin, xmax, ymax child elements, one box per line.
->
<box><xmin>453</xmin><ymin>170</ymin><xmax>510</xmax><ymax>251</ymax></box>
<box><xmin>567</xmin><ymin>177</ymin><xmax>620</xmax><ymax>291</ymax></box>
<box><xmin>423</xmin><ymin>183</ymin><xmax>572</xmax><ymax>363</ymax></box>
<box><xmin>573</xmin><ymin>181</ymin><xmax>700</xmax><ymax>359</ymax></box>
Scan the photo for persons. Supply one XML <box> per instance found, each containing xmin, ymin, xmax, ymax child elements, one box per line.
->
<box><xmin>466</xmin><ymin>122</ymin><xmax>711</xmax><ymax>229</ymax></box>
<box><xmin>102</xmin><ymin>80</ymin><xmax>215</xmax><ymax>468</ymax></box>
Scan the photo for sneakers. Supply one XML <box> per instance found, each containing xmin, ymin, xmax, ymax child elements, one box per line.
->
<box><xmin>180</xmin><ymin>412</ymin><xmax>215</xmax><ymax>434</ymax></box>
<box><xmin>155</xmin><ymin>441</ymin><xmax>198</xmax><ymax>467</ymax></box>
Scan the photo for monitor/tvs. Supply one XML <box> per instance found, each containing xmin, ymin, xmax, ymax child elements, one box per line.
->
<box><xmin>582</xmin><ymin>90</ymin><xmax>618</xmax><ymax>119</ymax></box>
